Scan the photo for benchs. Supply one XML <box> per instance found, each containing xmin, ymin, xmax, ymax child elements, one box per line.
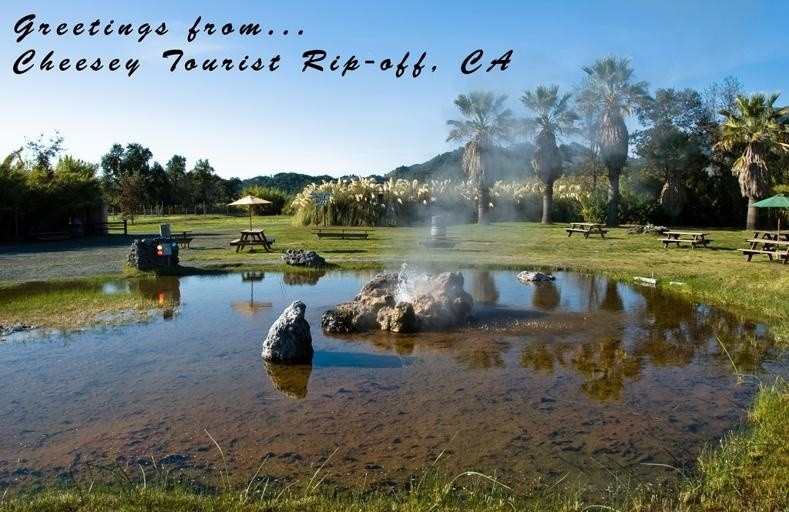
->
<box><xmin>230</xmin><ymin>239</ymin><xmax>241</xmax><ymax>252</ymax></box>
<box><xmin>661</xmin><ymin>238</ymin><xmax>710</xmax><ymax>250</ymax></box>
<box><xmin>566</xmin><ymin>228</ymin><xmax>610</xmax><ymax>239</ymax></box>
<box><xmin>266</xmin><ymin>238</ymin><xmax>275</xmax><ymax>252</ymax></box>
<box><xmin>737</xmin><ymin>249</ymin><xmax>789</xmax><ymax>264</ymax></box>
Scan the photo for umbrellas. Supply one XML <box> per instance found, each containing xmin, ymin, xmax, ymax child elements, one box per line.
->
<box><xmin>226</xmin><ymin>194</ymin><xmax>279</xmax><ymax>232</ymax></box>
<box><xmin>749</xmin><ymin>193</ymin><xmax>789</xmax><ymax>240</ymax></box>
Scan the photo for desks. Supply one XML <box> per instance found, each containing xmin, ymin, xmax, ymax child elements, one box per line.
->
<box><xmin>568</xmin><ymin>223</ymin><xmax>605</xmax><ymax>239</ymax></box>
<box><xmin>746</xmin><ymin>239</ymin><xmax>789</xmax><ymax>264</ymax></box>
<box><xmin>663</xmin><ymin>232</ymin><xmax>710</xmax><ymax>249</ymax></box>
<box><xmin>749</xmin><ymin>230</ymin><xmax>788</xmax><ymax>245</ymax></box>
<box><xmin>235</xmin><ymin>229</ymin><xmax>270</xmax><ymax>252</ymax></box>
